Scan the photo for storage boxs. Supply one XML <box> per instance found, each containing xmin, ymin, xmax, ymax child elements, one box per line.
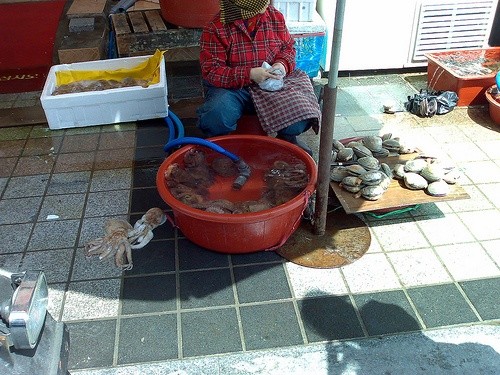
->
<box><xmin>40</xmin><ymin>52</ymin><xmax>170</xmax><ymax>131</ymax></box>
<box><xmin>425</xmin><ymin>44</ymin><xmax>500</xmax><ymax>107</ymax></box>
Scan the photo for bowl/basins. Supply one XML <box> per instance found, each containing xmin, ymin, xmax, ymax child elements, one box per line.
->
<box><xmin>155</xmin><ymin>135</ymin><xmax>318</xmax><ymax>253</ymax></box>
<box><xmin>484</xmin><ymin>85</ymin><xmax>500</xmax><ymax>125</ymax></box>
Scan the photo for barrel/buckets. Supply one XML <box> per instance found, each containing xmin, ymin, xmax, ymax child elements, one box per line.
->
<box><xmin>160</xmin><ymin>0</ymin><xmax>220</xmax><ymax>29</ymax></box>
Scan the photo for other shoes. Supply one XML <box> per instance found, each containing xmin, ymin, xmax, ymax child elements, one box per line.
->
<box><xmin>275</xmin><ymin>131</ymin><xmax>314</xmax><ymax>157</ymax></box>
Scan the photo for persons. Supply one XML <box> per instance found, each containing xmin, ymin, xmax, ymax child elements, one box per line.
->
<box><xmin>194</xmin><ymin>0</ymin><xmax>320</xmax><ymax>156</ymax></box>
